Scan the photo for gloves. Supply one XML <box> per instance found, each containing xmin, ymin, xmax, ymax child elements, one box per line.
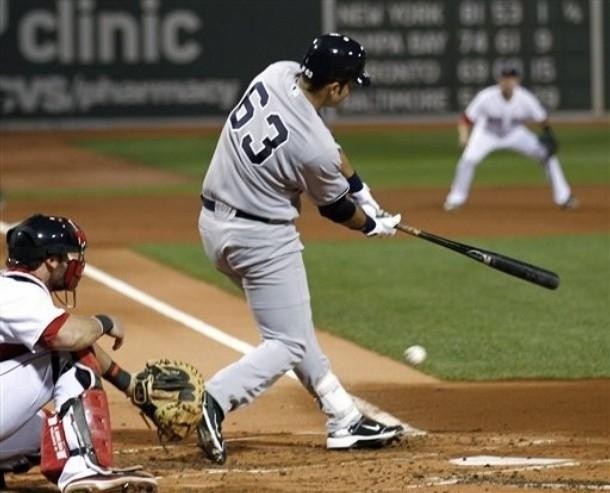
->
<box><xmin>350</xmin><ymin>181</ymin><xmax>402</xmax><ymax>238</ymax></box>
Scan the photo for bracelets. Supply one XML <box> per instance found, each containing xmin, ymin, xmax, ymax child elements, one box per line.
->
<box><xmin>101</xmin><ymin>359</ymin><xmax>131</xmax><ymax>392</ymax></box>
<box><xmin>91</xmin><ymin>313</ymin><xmax>113</xmax><ymax>335</ymax></box>
<box><xmin>346</xmin><ymin>170</ymin><xmax>363</xmax><ymax>193</ymax></box>
<box><xmin>350</xmin><ymin>213</ymin><xmax>376</xmax><ymax>233</ymax></box>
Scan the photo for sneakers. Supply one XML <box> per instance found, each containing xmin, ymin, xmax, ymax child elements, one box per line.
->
<box><xmin>559</xmin><ymin>195</ymin><xmax>582</xmax><ymax>213</ymax></box>
<box><xmin>197</xmin><ymin>391</ymin><xmax>225</xmax><ymax>466</ymax></box>
<box><xmin>445</xmin><ymin>199</ymin><xmax>458</xmax><ymax>213</ymax></box>
<box><xmin>325</xmin><ymin>414</ymin><xmax>405</xmax><ymax>451</ymax></box>
<box><xmin>63</xmin><ymin>471</ymin><xmax>158</xmax><ymax>493</ymax></box>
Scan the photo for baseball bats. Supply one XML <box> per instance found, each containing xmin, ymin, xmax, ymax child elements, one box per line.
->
<box><xmin>395</xmin><ymin>224</ymin><xmax>560</xmax><ymax>289</ymax></box>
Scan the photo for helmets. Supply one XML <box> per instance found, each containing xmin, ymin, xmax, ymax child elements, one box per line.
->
<box><xmin>302</xmin><ymin>33</ymin><xmax>372</xmax><ymax>89</ymax></box>
<box><xmin>4</xmin><ymin>212</ymin><xmax>87</xmax><ymax>310</ymax></box>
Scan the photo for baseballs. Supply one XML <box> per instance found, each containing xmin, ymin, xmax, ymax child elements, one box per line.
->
<box><xmin>405</xmin><ymin>345</ymin><xmax>425</xmax><ymax>364</ymax></box>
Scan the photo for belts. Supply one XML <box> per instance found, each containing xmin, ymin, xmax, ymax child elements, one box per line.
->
<box><xmin>200</xmin><ymin>195</ymin><xmax>288</xmax><ymax>223</ymax></box>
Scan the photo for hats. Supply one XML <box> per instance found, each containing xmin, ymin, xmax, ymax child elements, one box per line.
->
<box><xmin>500</xmin><ymin>66</ymin><xmax>521</xmax><ymax>78</ymax></box>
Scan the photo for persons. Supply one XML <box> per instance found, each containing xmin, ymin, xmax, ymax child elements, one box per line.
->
<box><xmin>0</xmin><ymin>213</ymin><xmax>204</xmax><ymax>493</ymax></box>
<box><xmin>196</xmin><ymin>31</ymin><xmax>405</xmax><ymax>463</ymax></box>
<box><xmin>441</xmin><ymin>66</ymin><xmax>578</xmax><ymax>210</ymax></box>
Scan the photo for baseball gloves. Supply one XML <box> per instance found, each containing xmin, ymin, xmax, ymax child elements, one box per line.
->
<box><xmin>132</xmin><ymin>359</ymin><xmax>205</xmax><ymax>453</ymax></box>
